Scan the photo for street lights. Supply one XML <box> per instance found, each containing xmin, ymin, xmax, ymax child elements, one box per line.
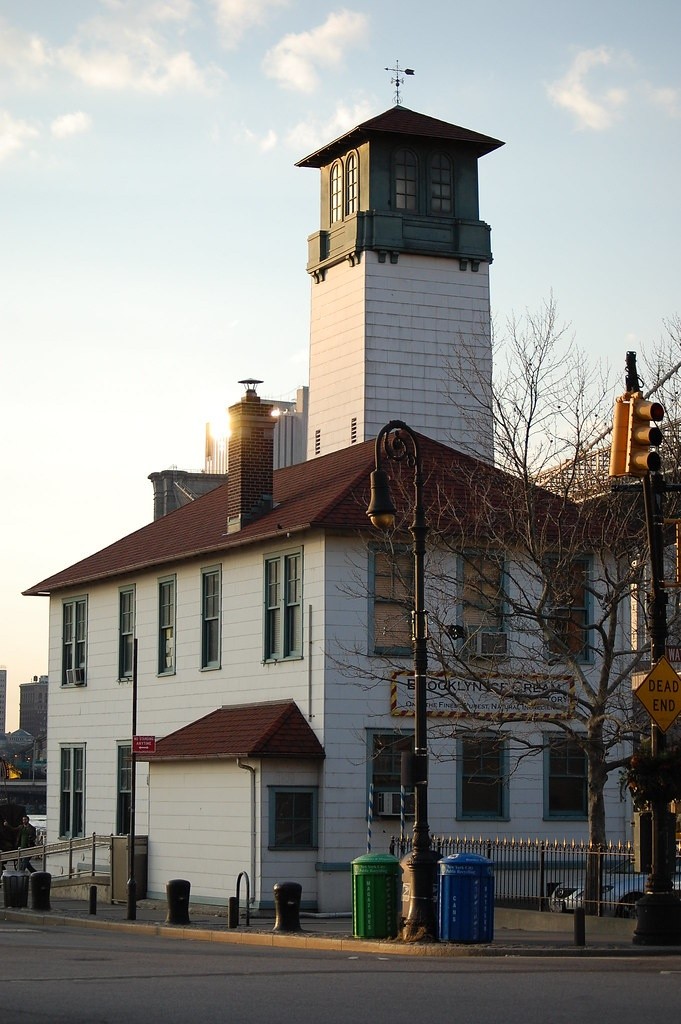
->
<box><xmin>366</xmin><ymin>420</ymin><xmax>439</xmax><ymax>945</ymax></box>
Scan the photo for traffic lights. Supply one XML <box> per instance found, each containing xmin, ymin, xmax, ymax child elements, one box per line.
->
<box><xmin>627</xmin><ymin>398</ymin><xmax>663</xmax><ymax>478</ymax></box>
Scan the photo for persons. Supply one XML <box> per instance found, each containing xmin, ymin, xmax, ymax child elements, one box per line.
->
<box><xmin>4</xmin><ymin>816</ymin><xmax>37</xmax><ymax>873</ymax></box>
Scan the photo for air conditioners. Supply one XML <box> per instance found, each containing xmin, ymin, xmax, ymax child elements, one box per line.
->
<box><xmin>476</xmin><ymin>631</ymin><xmax>508</xmax><ymax>653</ymax></box>
<box><xmin>67</xmin><ymin>667</ymin><xmax>84</xmax><ymax>684</ymax></box>
<box><xmin>377</xmin><ymin>791</ymin><xmax>418</xmax><ymax>816</ymax></box>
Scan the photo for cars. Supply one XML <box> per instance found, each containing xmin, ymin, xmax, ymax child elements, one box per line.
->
<box><xmin>549</xmin><ymin>857</ymin><xmax>681</xmax><ymax>919</ymax></box>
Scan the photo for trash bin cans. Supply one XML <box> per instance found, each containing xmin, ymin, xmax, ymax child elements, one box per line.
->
<box><xmin>438</xmin><ymin>853</ymin><xmax>496</xmax><ymax>944</ymax></box>
<box><xmin>351</xmin><ymin>853</ymin><xmax>404</xmax><ymax>939</ymax></box>
